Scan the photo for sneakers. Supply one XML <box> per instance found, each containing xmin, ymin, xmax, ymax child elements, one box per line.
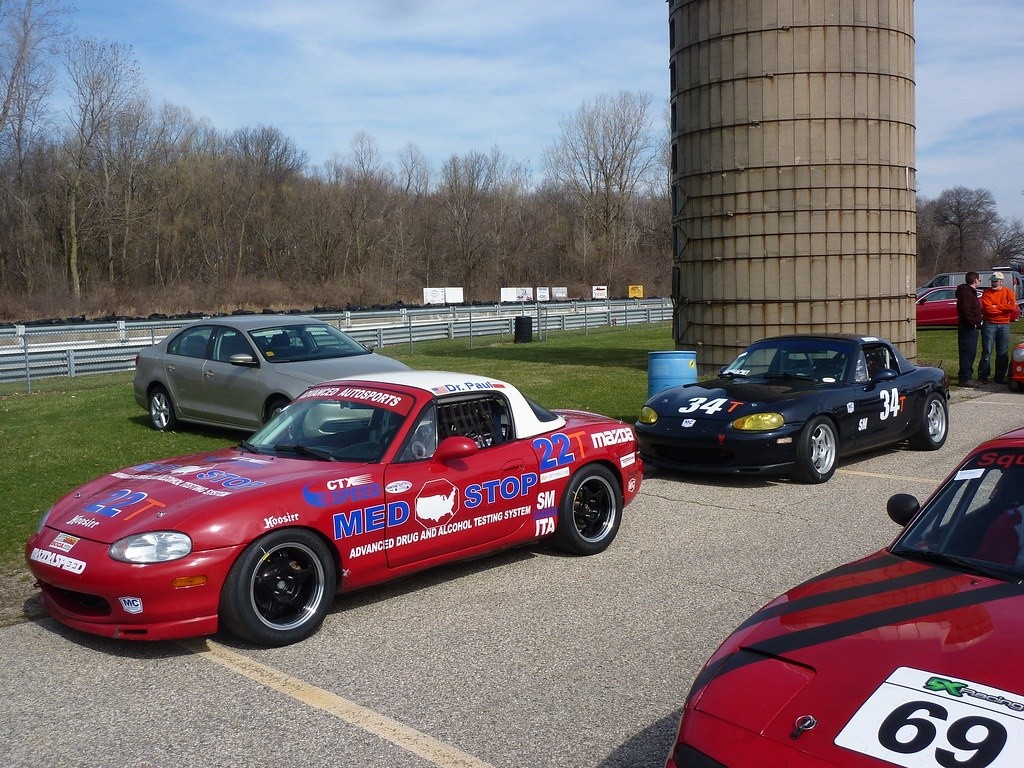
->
<box><xmin>958</xmin><ymin>379</ymin><xmax>980</xmax><ymax>388</ymax></box>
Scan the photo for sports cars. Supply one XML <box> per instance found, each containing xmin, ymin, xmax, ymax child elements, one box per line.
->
<box><xmin>22</xmin><ymin>367</ymin><xmax>644</xmax><ymax>648</ymax></box>
<box><xmin>634</xmin><ymin>334</ymin><xmax>952</xmax><ymax>485</ymax></box>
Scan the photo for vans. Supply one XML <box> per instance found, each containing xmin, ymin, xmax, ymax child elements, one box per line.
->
<box><xmin>916</xmin><ymin>285</ymin><xmax>1021</xmax><ymax>330</ymax></box>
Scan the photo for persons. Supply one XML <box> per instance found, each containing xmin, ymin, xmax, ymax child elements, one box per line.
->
<box><xmin>978</xmin><ymin>271</ymin><xmax>1015</xmax><ymax>385</ymax></box>
<box><xmin>955</xmin><ymin>272</ymin><xmax>982</xmax><ymax>388</ymax></box>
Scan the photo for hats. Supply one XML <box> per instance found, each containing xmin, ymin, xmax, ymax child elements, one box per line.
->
<box><xmin>988</xmin><ymin>271</ymin><xmax>1005</xmax><ymax>282</ymax></box>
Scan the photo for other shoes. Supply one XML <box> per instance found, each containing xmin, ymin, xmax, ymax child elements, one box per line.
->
<box><xmin>995</xmin><ymin>380</ymin><xmax>1007</xmax><ymax>384</ymax></box>
<box><xmin>978</xmin><ymin>377</ymin><xmax>988</xmax><ymax>384</ymax></box>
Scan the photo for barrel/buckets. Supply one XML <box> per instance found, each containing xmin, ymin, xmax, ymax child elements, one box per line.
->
<box><xmin>515</xmin><ymin>315</ymin><xmax>532</xmax><ymax>343</ymax></box>
<box><xmin>646</xmin><ymin>350</ymin><xmax>698</xmax><ymax>400</ymax></box>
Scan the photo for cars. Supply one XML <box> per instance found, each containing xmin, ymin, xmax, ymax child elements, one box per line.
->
<box><xmin>1007</xmin><ymin>341</ymin><xmax>1024</xmax><ymax>392</ymax></box>
<box><xmin>923</xmin><ymin>265</ymin><xmax>1024</xmax><ymax>313</ymax></box>
<box><xmin>132</xmin><ymin>315</ymin><xmax>415</xmax><ymax>442</ymax></box>
<box><xmin>663</xmin><ymin>423</ymin><xmax>1024</xmax><ymax>768</ymax></box>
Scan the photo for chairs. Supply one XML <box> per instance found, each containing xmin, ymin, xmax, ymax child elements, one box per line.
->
<box><xmin>185</xmin><ymin>335</ymin><xmax>207</xmax><ymax>358</ymax></box>
<box><xmin>976</xmin><ymin>504</ymin><xmax>1024</xmax><ymax>565</ymax></box>
<box><xmin>270</xmin><ymin>331</ymin><xmax>292</xmax><ymax>357</ymax></box>
